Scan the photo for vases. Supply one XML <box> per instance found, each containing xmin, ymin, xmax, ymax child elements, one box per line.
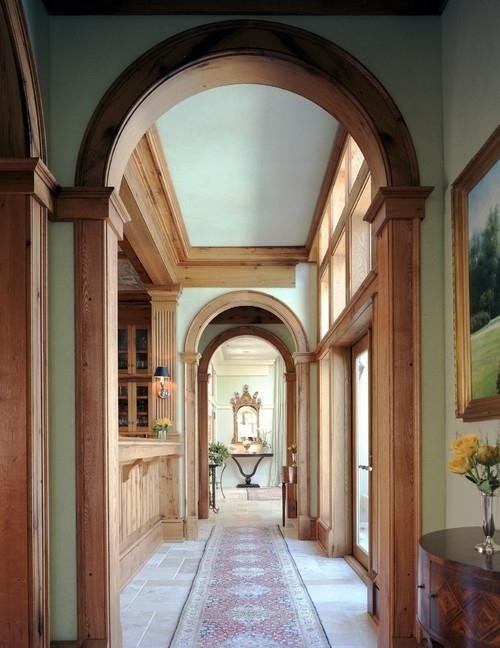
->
<box><xmin>476</xmin><ymin>491</ymin><xmax>500</xmax><ymax>554</ymax></box>
<box><xmin>292</xmin><ymin>453</ymin><xmax>297</xmax><ymax>468</ymax></box>
<box><xmin>157</xmin><ymin>431</ymin><xmax>167</xmax><ymax>440</ymax></box>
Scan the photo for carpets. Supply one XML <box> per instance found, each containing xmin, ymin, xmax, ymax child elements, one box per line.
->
<box><xmin>247</xmin><ymin>486</ymin><xmax>283</xmax><ymax>501</ymax></box>
<box><xmin>168</xmin><ymin>523</ymin><xmax>332</xmax><ymax>648</ymax></box>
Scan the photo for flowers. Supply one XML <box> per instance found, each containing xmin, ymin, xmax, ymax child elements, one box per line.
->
<box><xmin>152</xmin><ymin>417</ymin><xmax>173</xmax><ymax>431</ymax></box>
<box><xmin>288</xmin><ymin>443</ymin><xmax>297</xmax><ymax>461</ymax></box>
<box><xmin>448</xmin><ymin>432</ymin><xmax>500</xmax><ymax>533</ymax></box>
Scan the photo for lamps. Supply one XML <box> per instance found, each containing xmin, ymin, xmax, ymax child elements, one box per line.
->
<box><xmin>154</xmin><ymin>366</ymin><xmax>171</xmax><ymax>398</ymax></box>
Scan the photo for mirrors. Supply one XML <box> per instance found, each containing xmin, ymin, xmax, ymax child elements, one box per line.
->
<box><xmin>231</xmin><ymin>384</ymin><xmax>263</xmax><ymax>444</ymax></box>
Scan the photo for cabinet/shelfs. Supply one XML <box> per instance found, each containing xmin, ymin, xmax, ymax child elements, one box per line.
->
<box><xmin>118</xmin><ymin>325</ymin><xmax>152</xmax><ymax>439</ymax></box>
<box><xmin>209</xmin><ymin>463</ymin><xmax>219</xmax><ymax>513</ymax></box>
<box><xmin>415</xmin><ymin>526</ymin><xmax>500</xmax><ymax>647</ymax></box>
<box><xmin>280</xmin><ymin>465</ymin><xmax>297</xmax><ymax>487</ymax></box>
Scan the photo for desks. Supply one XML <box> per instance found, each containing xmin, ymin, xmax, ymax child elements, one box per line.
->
<box><xmin>227</xmin><ymin>453</ymin><xmax>273</xmax><ymax>488</ymax></box>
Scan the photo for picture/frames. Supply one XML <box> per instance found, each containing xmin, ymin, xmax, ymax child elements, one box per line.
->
<box><xmin>450</xmin><ymin>121</ymin><xmax>500</xmax><ymax>423</ymax></box>
